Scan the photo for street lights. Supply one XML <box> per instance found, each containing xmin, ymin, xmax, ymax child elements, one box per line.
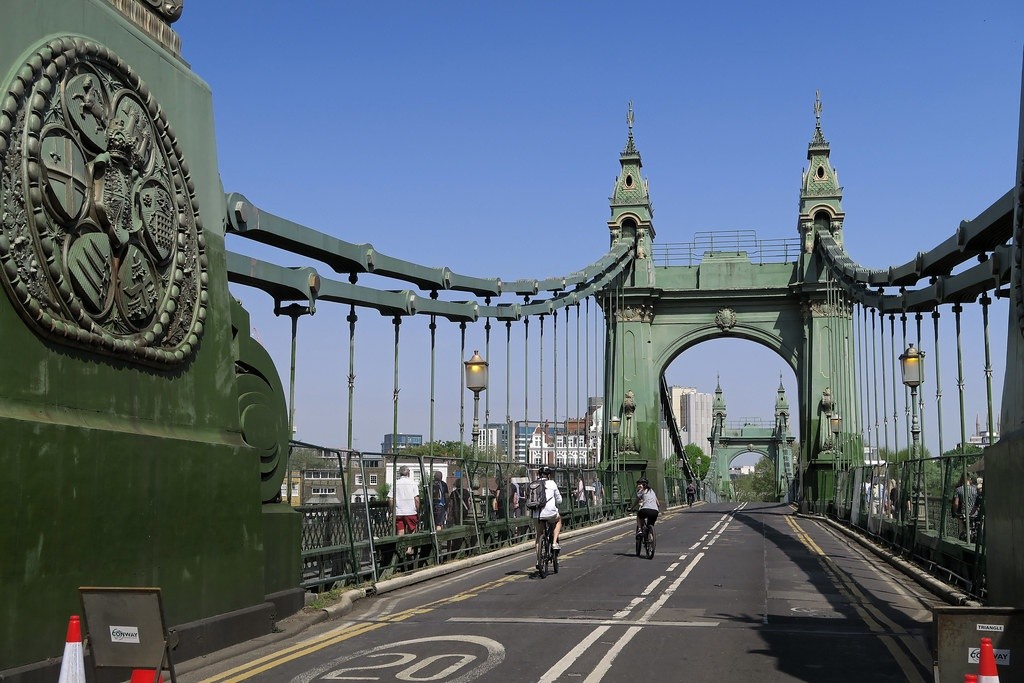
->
<box><xmin>463</xmin><ymin>350</ymin><xmax>491</xmax><ymax>520</ymax></box>
<box><xmin>830</xmin><ymin>412</ymin><xmax>843</xmax><ymax>505</ymax></box>
<box><xmin>607</xmin><ymin>415</ymin><xmax>621</xmax><ymax>501</ymax></box>
<box><xmin>898</xmin><ymin>342</ymin><xmax>933</xmax><ymax>530</ymax></box>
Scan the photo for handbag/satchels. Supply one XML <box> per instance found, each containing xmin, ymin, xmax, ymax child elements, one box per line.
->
<box><xmin>493</xmin><ymin>498</ymin><xmax>498</xmax><ymax>510</ymax></box>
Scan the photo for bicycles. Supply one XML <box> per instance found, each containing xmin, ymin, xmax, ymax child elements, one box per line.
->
<box><xmin>957</xmin><ymin>513</ymin><xmax>984</xmax><ymax>543</ymax></box>
<box><xmin>687</xmin><ymin>494</ymin><xmax>694</xmax><ymax>508</ymax></box>
<box><xmin>536</xmin><ymin>518</ymin><xmax>560</xmax><ymax>578</ymax></box>
<box><xmin>635</xmin><ymin>516</ymin><xmax>659</xmax><ymax>558</ymax></box>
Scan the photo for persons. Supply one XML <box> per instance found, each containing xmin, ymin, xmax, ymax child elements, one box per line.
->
<box><xmin>686</xmin><ymin>482</ymin><xmax>696</xmax><ymax>505</ymax></box>
<box><xmin>862</xmin><ymin>474</ymin><xmax>910</xmax><ymax>521</ymax></box>
<box><xmin>952</xmin><ymin>472</ymin><xmax>984</xmax><ymax>545</ymax></box>
<box><xmin>571</xmin><ymin>474</ymin><xmax>586</xmax><ymax>508</ymax></box>
<box><xmin>531</xmin><ymin>466</ymin><xmax>561</xmax><ymax>565</ymax></box>
<box><xmin>627</xmin><ymin>477</ymin><xmax>660</xmax><ymax>542</ymax></box>
<box><xmin>496</xmin><ymin>473</ymin><xmax>527</xmax><ymax>518</ymax></box>
<box><xmin>426</xmin><ymin>471</ymin><xmax>450</xmax><ymax>530</ymax></box>
<box><xmin>387</xmin><ymin>465</ymin><xmax>420</xmax><ymax>560</ymax></box>
<box><xmin>591</xmin><ymin>476</ymin><xmax>605</xmax><ymax>519</ymax></box>
<box><xmin>450</xmin><ymin>479</ymin><xmax>470</xmax><ymax>524</ymax></box>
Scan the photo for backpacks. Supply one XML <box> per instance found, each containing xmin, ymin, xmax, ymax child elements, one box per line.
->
<box><xmin>525</xmin><ymin>479</ymin><xmax>552</xmax><ymax>509</ymax></box>
<box><xmin>428</xmin><ymin>481</ymin><xmax>445</xmax><ymax>509</ymax></box>
<box><xmin>688</xmin><ymin>487</ymin><xmax>693</xmax><ymax>491</ymax></box>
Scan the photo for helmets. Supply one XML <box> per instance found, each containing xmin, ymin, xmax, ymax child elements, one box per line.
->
<box><xmin>637</xmin><ymin>477</ymin><xmax>649</xmax><ymax>483</ymax></box>
<box><xmin>537</xmin><ymin>466</ymin><xmax>551</xmax><ymax>476</ymax></box>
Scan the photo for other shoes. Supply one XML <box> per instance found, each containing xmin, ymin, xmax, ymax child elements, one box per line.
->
<box><xmin>536</xmin><ymin>560</ymin><xmax>544</xmax><ymax>569</ymax></box>
<box><xmin>635</xmin><ymin>530</ymin><xmax>642</xmax><ymax>540</ymax></box>
<box><xmin>552</xmin><ymin>543</ymin><xmax>561</xmax><ymax>549</ymax></box>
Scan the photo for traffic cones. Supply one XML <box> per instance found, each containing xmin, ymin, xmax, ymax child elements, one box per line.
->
<box><xmin>130</xmin><ymin>668</ymin><xmax>163</xmax><ymax>683</ymax></box>
<box><xmin>58</xmin><ymin>614</ymin><xmax>88</xmax><ymax>683</ymax></box>
<box><xmin>977</xmin><ymin>637</ymin><xmax>1001</xmax><ymax>683</ymax></box>
